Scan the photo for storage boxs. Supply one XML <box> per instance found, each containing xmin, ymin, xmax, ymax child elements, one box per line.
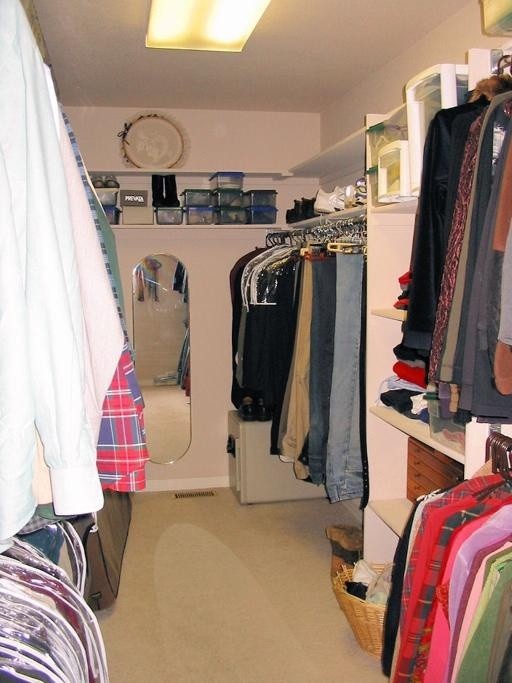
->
<box><xmin>89</xmin><ymin>172</ymin><xmax>279</xmax><ymax>225</ymax></box>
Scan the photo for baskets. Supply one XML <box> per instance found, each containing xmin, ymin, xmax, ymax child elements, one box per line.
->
<box><xmin>330</xmin><ymin>559</ymin><xmax>393</xmax><ymax>660</ymax></box>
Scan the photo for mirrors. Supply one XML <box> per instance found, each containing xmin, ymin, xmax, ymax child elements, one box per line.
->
<box><xmin>129</xmin><ymin>254</ymin><xmax>192</xmax><ymax>466</ymax></box>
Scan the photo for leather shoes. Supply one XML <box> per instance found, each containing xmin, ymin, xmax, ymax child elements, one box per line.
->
<box><xmin>283</xmin><ymin>196</ymin><xmax>318</xmax><ymax>223</ymax></box>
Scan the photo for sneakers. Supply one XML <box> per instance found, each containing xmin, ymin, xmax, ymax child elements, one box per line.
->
<box><xmin>353</xmin><ymin>175</ymin><xmax>367</xmax><ymax>206</ymax></box>
<box><xmin>343</xmin><ymin>185</ymin><xmax>356</xmax><ymax>209</ymax></box>
<box><xmin>328</xmin><ymin>185</ymin><xmax>345</xmax><ymax>212</ymax></box>
<box><xmin>313</xmin><ymin>186</ymin><xmax>344</xmax><ymax>214</ymax></box>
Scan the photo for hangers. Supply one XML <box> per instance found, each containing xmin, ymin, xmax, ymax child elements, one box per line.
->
<box><xmin>266</xmin><ymin>214</ymin><xmax>366</xmax><ymax>254</ymax></box>
<box><xmin>491</xmin><ymin>56</ymin><xmax>512</xmax><ymax>79</ymax></box>
<box><xmin>486</xmin><ymin>434</ymin><xmax>512</xmax><ymax>499</ymax></box>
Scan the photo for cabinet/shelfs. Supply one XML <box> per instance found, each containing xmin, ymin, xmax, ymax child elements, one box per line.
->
<box><xmin>279</xmin><ymin>105</ymin><xmax>512</xmax><ymax>569</ymax></box>
<box><xmin>407</xmin><ymin>439</ymin><xmax>462</xmax><ymax>506</ymax></box>
